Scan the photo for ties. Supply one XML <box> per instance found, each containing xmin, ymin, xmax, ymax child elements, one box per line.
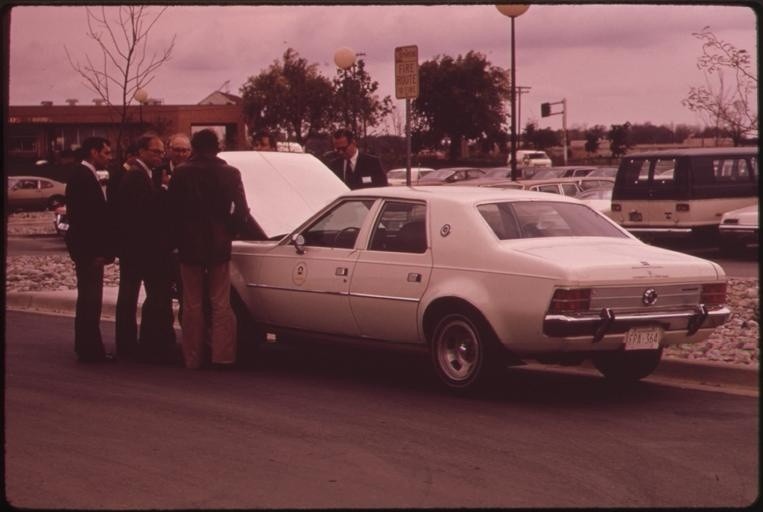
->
<box><xmin>345</xmin><ymin>161</ymin><xmax>353</xmax><ymax>183</ymax></box>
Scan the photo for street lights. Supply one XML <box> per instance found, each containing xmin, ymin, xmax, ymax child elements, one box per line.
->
<box><xmin>490</xmin><ymin>3</ymin><xmax>528</xmax><ymax>176</ymax></box>
<box><xmin>333</xmin><ymin>48</ymin><xmax>358</xmax><ymax>149</ymax></box>
<box><xmin>133</xmin><ymin>83</ymin><xmax>149</xmax><ymax>131</ymax></box>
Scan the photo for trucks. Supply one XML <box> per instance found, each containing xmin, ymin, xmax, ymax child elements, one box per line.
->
<box><xmin>506</xmin><ymin>147</ymin><xmax>552</xmax><ymax>166</ymax></box>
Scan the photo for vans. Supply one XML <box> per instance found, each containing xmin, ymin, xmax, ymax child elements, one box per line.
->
<box><xmin>605</xmin><ymin>148</ymin><xmax>757</xmax><ymax>245</ymax></box>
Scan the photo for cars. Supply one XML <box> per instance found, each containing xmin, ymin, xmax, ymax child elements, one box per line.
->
<box><xmin>270</xmin><ymin>139</ymin><xmax>305</xmax><ymax>154</ymax></box>
<box><xmin>351</xmin><ymin>158</ymin><xmax>746</xmax><ymax>250</ymax></box>
<box><xmin>719</xmin><ymin>202</ymin><xmax>759</xmax><ymax>245</ymax></box>
<box><xmin>6</xmin><ymin>174</ymin><xmax>69</xmax><ymax>214</ymax></box>
<box><xmin>174</xmin><ymin>140</ymin><xmax>733</xmax><ymax>391</ymax></box>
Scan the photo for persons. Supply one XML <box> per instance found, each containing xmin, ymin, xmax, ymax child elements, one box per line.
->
<box><xmin>58</xmin><ymin>130</ymin><xmax>248</xmax><ymax>369</ymax></box>
<box><xmin>522</xmin><ymin>153</ymin><xmax>531</xmax><ymax>165</ymax></box>
<box><xmin>329</xmin><ymin>128</ymin><xmax>387</xmax><ymax>190</ymax></box>
